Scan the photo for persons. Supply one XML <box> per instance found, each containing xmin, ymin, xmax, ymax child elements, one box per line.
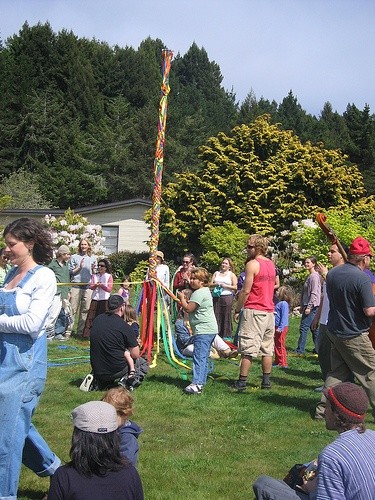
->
<box><xmin>101</xmin><ymin>386</ymin><xmax>143</xmax><ymax>467</ymax></box>
<box><xmin>253</xmin><ymin>381</ymin><xmax>375</xmax><ymax>500</ymax></box>
<box><xmin>0</xmin><ymin>234</ymin><xmax>375</xmax><ymax>421</ymax></box>
<box><xmin>0</xmin><ymin>218</ymin><xmax>63</xmax><ymax>500</ymax></box>
<box><xmin>47</xmin><ymin>401</ymin><xmax>144</xmax><ymax>500</ymax></box>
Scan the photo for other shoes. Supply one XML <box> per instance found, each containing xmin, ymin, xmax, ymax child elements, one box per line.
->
<box><xmin>65</xmin><ymin>334</ymin><xmax>69</xmax><ymax>339</ymax></box>
<box><xmin>233</xmin><ymin>380</ymin><xmax>247</xmax><ymax>389</ymax></box>
<box><xmin>42</xmin><ymin>475</ymin><xmax>52</xmax><ymax>500</ymax></box>
<box><xmin>118</xmin><ymin>378</ymin><xmax>136</xmax><ymax>393</ymax></box>
<box><xmin>55</xmin><ymin>334</ymin><xmax>65</xmax><ymax>341</ymax></box>
<box><xmin>188</xmin><ymin>374</ymin><xmax>193</xmax><ymax>383</ymax></box>
<box><xmin>218</xmin><ymin>349</ymin><xmax>238</xmax><ymax>359</ymax></box>
<box><xmin>79</xmin><ymin>373</ymin><xmax>95</xmax><ymax>392</ymax></box>
<box><xmin>261</xmin><ymin>382</ymin><xmax>270</xmax><ymax>389</ymax></box>
<box><xmin>77</xmin><ymin>334</ymin><xmax>83</xmax><ymax>338</ymax></box>
<box><xmin>182</xmin><ymin>383</ymin><xmax>202</xmax><ymax>394</ymax></box>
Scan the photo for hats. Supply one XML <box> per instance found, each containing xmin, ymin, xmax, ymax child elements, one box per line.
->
<box><xmin>322</xmin><ymin>382</ymin><xmax>368</xmax><ymax>422</ymax></box>
<box><xmin>71</xmin><ymin>401</ymin><xmax>118</xmax><ymax>434</ymax></box>
<box><xmin>108</xmin><ymin>295</ymin><xmax>127</xmax><ymax>310</ymax></box>
<box><xmin>349</xmin><ymin>236</ymin><xmax>374</xmax><ymax>257</ymax></box>
<box><xmin>156</xmin><ymin>251</ymin><xmax>164</xmax><ymax>262</ymax></box>
<box><xmin>58</xmin><ymin>244</ymin><xmax>71</xmax><ymax>255</ymax></box>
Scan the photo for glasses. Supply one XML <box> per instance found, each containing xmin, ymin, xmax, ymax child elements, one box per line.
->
<box><xmin>363</xmin><ymin>254</ymin><xmax>372</xmax><ymax>259</ymax></box>
<box><xmin>245</xmin><ymin>244</ymin><xmax>255</xmax><ymax>249</ymax></box>
<box><xmin>182</xmin><ymin>261</ymin><xmax>191</xmax><ymax>264</ymax></box>
<box><xmin>97</xmin><ymin>265</ymin><xmax>105</xmax><ymax>268</ymax></box>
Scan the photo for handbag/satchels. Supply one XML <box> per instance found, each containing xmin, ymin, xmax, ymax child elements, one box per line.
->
<box><xmin>212</xmin><ymin>285</ymin><xmax>224</xmax><ymax>297</ymax></box>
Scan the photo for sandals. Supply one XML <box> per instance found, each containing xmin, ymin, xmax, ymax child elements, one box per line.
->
<box><xmin>128</xmin><ymin>370</ymin><xmax>137</xmax><ymax>379</ymax></box>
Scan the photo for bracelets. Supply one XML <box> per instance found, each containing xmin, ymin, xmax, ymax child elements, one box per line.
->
<box><xmin>235</xmin><ymin>309</ymin><xmax>241</xmax><ymax>313</ymax></box>
